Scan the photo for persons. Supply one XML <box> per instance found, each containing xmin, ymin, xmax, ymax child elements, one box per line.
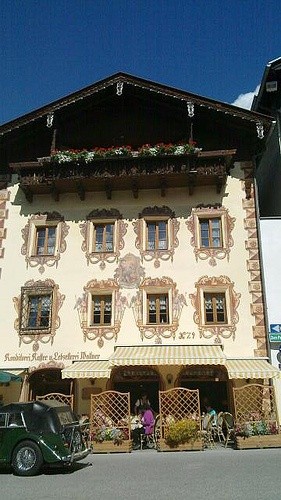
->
<box><xmin>130</xmin><ymin>392</ymin><xmax>157</xmax><ymax>450</ymax></box>
<box><xmin>91</xmin><ymin>407</ymin><xmax>109</xmax><ymax>427</ymax></box>
<box><xmin>205</xmin><ymin>404</ymin><xmax>218</xmax><ymax>439</ymax></box>
<box><xmin>78</xmin><ymin>413</ymin><xmax>90</xmax><ymax>450</ymax></box>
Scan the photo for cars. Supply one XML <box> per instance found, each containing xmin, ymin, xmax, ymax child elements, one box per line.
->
<box><xmin>0</xmin><ymin>398</ymin><xmax>92</xmax><ymax>477</ymax></box>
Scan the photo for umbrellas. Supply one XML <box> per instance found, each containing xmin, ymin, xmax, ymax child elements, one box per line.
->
<box><xmin>0</xmin><ymin>369</ymin><xmax>23</xmax><ymax>387</ymax></box>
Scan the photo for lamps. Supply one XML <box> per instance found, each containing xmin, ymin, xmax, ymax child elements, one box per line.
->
<box><xmin>167</xmin><ymin>374</ymin><xmax>173</xmax><ymax>384</ymax></box>
<box><xmin>88</xmin><ymin>378</ymin><xmax>95</xmax><ymax>385</ymax></box>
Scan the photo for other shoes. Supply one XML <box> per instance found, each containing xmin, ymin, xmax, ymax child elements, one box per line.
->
<box><xmin>134</xmin><ymin>444</ymin><xmax>143</xmax><ymax>449</ymax></box>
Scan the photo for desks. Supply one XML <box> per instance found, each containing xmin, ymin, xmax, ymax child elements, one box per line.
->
<box><xmin>106</xmin><ymin>426</ymin><xmax>129</xmax><ymax>430</ymax></box>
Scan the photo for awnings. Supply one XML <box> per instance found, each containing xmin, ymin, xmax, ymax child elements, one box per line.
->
<box><xmin>224</xmin><ymin>357</ymin><xmax>281</xmax><ymax>380</ymax></box>
<box><xmin>61</xmin><ymin>361</ymin><xmax>113</xmax><ymax>379</ymax></box>
<box><xmin>0</xmin><ymin>367</ymin><xmax>28</xmax><ymax>375</ymax></box>
<box><xmin>107</xmin><ymin>346</ymin><xmax>227</xmax><ymax>366</ymax></box>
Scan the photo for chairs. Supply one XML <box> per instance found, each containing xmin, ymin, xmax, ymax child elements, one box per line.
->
<box><xmin>212</xmin><ymin>411</ymin><xmax>226</xmax><ymax>442</ymax></box>
<box><xmin>196</xmin><ymin>413</ymin><xmax>216</xmax><ymax>449</ymax></box>
<box><xmin>222</xmin><ymin>411</ymin><xmax>236</xmax><ymax>448</ymax></box>
<box><xmin>140</xmin><ymin>413</ymin><xmax>163</xmax><ymax>451</ymax></box>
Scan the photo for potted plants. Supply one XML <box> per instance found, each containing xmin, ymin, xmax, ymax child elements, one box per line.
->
<box><xmin>236</xmin><ymin>420</ymin><xmax>281</xmax><ymax>449</ymax></box>
<box><xmin>86</xmin><ymin>428</ymin><xmax>133</xmax><ymax>453</ymax></box>
<box><xmin>156</xmin><ymin>418</ymin><xmax>206</xmax><ymax>451</ymax></box>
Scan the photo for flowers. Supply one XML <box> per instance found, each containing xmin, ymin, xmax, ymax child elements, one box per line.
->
<box><xmin>51</xmin><ymin>138</ymin><xmax>197</xmax><ymax>167</ymax></box>
